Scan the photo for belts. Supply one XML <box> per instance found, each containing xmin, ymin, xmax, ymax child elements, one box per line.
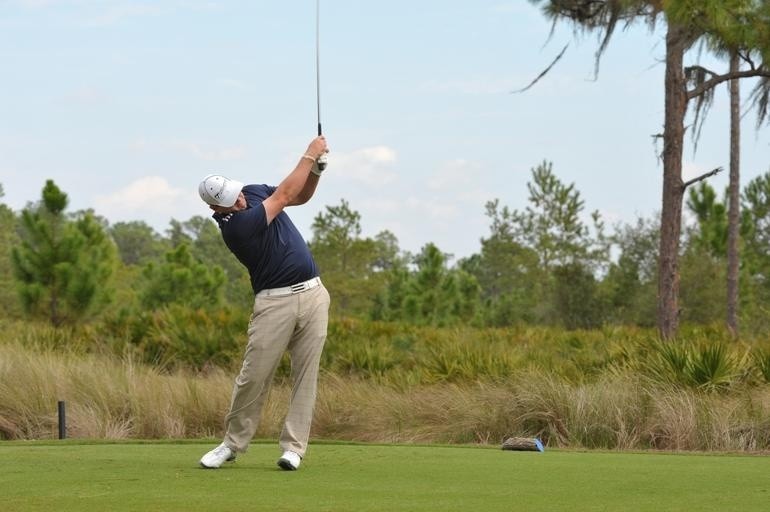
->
<box><xmin>262</xmin><ymin>275</ymin><xmax>320</xmax><ymax>296</ymax></box>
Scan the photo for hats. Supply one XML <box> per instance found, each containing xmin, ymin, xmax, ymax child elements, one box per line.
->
<box><xmin>198</xmin><ymin>174</ymin><xmax>244</xmax><ymax>207</ymax></box>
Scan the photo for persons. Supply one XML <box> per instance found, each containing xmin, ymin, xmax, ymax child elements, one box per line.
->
<box><xmin>197</xmin><ymin>134</ymin><xmax>332</xmax><ymax>472</ymax></box>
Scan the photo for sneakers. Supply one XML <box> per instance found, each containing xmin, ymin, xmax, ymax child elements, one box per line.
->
<box><xmin>277</xmin><ymin>450</ymin><xmax>301</xmax><ymax>471</ymax></box>
<box><xmin>200</xmin><ymin>442</ymin><xmax>237</xmax><ymax>468</ymax></box>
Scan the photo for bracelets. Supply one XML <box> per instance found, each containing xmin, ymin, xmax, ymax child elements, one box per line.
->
<box><xmin>301</xmin><ymin>154</ymin><xmax>316</xmax><ymax>163</ymax></box>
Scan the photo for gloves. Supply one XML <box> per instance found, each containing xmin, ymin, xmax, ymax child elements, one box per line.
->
<box><xmin>311</xmin><ymin>153</ymin><xmax>328</xmax><ymax>175</ymax></box>
